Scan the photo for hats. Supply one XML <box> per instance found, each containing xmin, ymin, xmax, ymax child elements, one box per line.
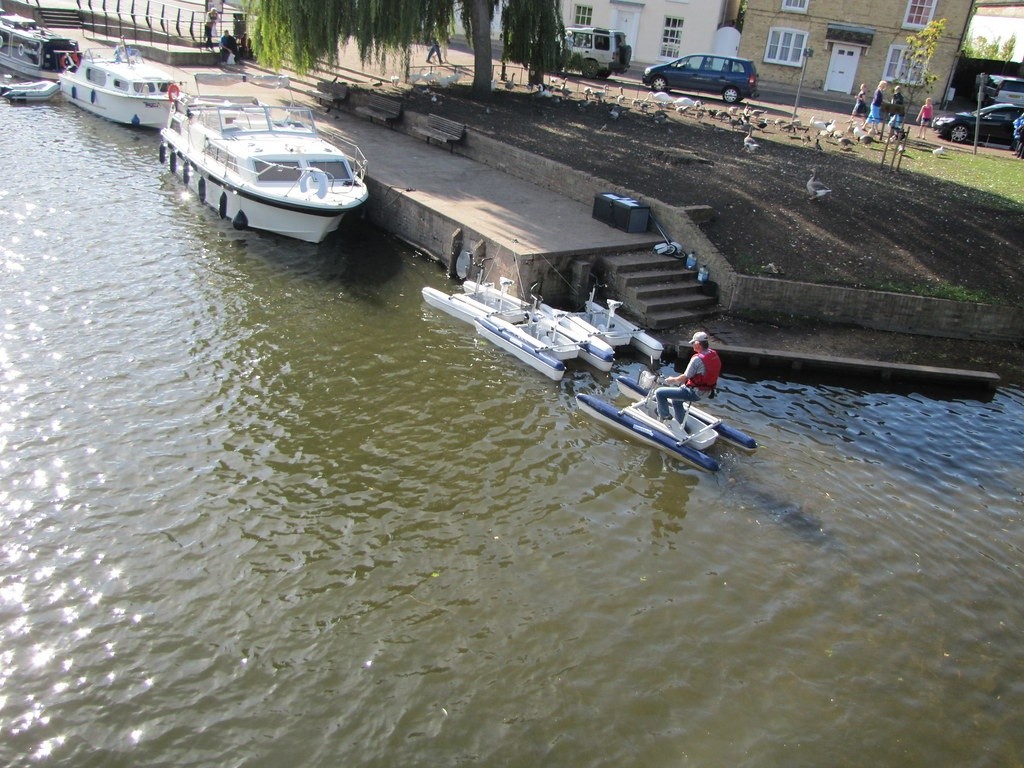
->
<box><xmin>688</xmin><ymin>332</ymin><xmax>708</xmax><ymax>343</ymax></box>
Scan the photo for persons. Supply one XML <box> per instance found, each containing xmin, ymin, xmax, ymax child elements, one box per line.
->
<box><xmin>426</xmin><ymin>36</ymin><xmax>445</xmax><ymax>64</ymax></box>
<box><xmin>205</xmin><ymin>8</ymin><xmax>217</xmax><ymax>52</ymax></box>
<box><xmin>1013</xmin><ymin>114</ymin><xmax>1024</xmax><ymax>159</ymax></box>
<box><xmin>916</xmin><ymin>98</ymin><xmax>933</xmax><ymax>140</ymax></box>
<box><xmin>862</xmin><ymin>80</ymin><xmax>887</xmax><ymax>135</ymax></box>
<box><xmin>889</xmin><ymin>86</ymin><xmax>906</xmax><ymax>140</ymax></box>
<box><xmin>655</xmin><ymin>332</ymin><xmax>721</xmax><ymax>431</ymax></box>
<box><xmin>219</xmin><ymin>30</ymin><xmax>240</xmax><ymax>65</ymax></box>
<box><xmin>847</xmin><ymin>84</ymin><xmax>867</xmax><ymax>123</ymax></box>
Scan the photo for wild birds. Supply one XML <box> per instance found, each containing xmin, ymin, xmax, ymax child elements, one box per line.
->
<box><xmin>423</xmin><ymin>71</ymin><xmax>466</xmax><ymax>88</ymax></box>
<box><xmin>673</xmin><ymin>97</ymin><xmax>906</xmax><ymax>154</ymax></box>
<box><xmin>526</xmin><ymin>76</ymin><xmax>573</xmax><ymax>98</ymax></box>
<box><xmin>807</xmin><ymin>168</ymin><xmax>833</xmax><ymax>200</ymax></box>
<box><xmin>391</xmin><ymin>76</ymin><xmax>400</xmax><ymax>87</ymax></box>
<box><xmin>505</xmin><ymin>73</ymin><xmax>516</xmax><ymax>91</ymax></box>
<box><xmin>743</xmin><ymin>126</ymin><xmax>762</xmax><ymax>151</ymax></box>
<box><xmin>686</xmin><ymin>252</ymin><xmax>697</xmax><ymax>269</ymax></box>
<box><xmin>645</xmin><ymin>91</ymin><xmax>673</xmax><ymax>104</ymax></box>
<box><xmin>933</xmin><ymin>146</ymin><xmax>944</xmax><ymax>158</ymax></box>
<box><xmin>409</xmin><ymin>68</ymin><xmax>426</xmax><ymax>85</ymax></box>
<box><xmin>584</xmin><ymin>84</ymin><xmax>673</xmax><ymax>121</ymax></box>
<box><xmin>698</xmin><ymin>264</ymin><xmax>709</xmax><ymax>282</ymax></box>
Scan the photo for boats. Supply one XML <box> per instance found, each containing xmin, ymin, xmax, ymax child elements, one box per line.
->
<box><xmin>540</xmin><ymin>297</ymin><xmax>665</xmax><ymax>373</ymax></box>
<box><xmin>575</xmin><ymin>369</ymin><xmax>759</xmax><ymax>474</ymax></box>
<box><xmin>59</xmin><ymin>42</ymin><xmax>188</xmax><ymax>129</ymax></box>
<box><xmin>158</xmin><ymin>70</ymin><xmax>369</xmax><ymax>243</ymax></box>
<box><xmin>0</xmin><ymin>80</ymin><xmax>59</xmax><ymax>101</ymax></box>
<box><xmin>420</xmin><ymin>239</ymin><xmax>616</xmax><ymax>381</ymax></box>
<box><xmin>0</xmin><ymin>8</ymin><xmax>82</xmax><ymax>82</ymax></box>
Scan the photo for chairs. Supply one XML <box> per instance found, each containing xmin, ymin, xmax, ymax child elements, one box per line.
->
<box><xmin>708</xmin><ymin>60</ymin><xmax>712</xmax><ymax>70</ymax></box>
<box><xmin>721</xmin><ymin>63</ymin><xmax>727</xmax><ymax>71</ymax></box>
<box><xmin>704</xmin><ymin>60</ymin><xmax>709</xmax><ymax>70</ymax></box>
<box><xmin>733</xmin><ymin>64</ymin><xmax>740</xmax><ymax>72</ymax></box>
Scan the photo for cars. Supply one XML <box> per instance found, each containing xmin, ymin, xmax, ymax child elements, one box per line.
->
<box><xmin>642</xmin><ymin>54</ymin><xmax>759</xmax><ymax>103</ymax></box>
<box><xmin>931</xmin><ymin>103</ymin><xmax>1024</xmax><ymax>143</ymax></box>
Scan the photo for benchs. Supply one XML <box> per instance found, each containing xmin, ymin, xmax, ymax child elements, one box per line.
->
<box><xmin>305</xmin><ymin>81</ymin><xmax>347</xmax><ymax>112</ymax></box>
<box><xmin>412</xmin><ymin>113</ymin><xmax>466</xmax><ymax>155</ymax></box>
<box><xmin>355</xmin><ymin>93</ymin><xmax>402</xmax><ymax>130</ymax></box>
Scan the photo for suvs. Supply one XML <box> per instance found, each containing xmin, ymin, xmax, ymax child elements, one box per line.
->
<box><xmin>556</xmin><ymin>23</ymin><xmax>631</xmax><ymax>78</ymax></box>
<box><xmin>984</xmin><ymin>75</ymin><xmax>1024</xmax><ymax>106</ymax></box>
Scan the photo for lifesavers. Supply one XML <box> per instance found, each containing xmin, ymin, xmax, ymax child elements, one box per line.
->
<box><xmin>168</xmin><ymin>84</ymin><xmax>180</xmax><ymax>103</ymax></box>
<box><xmin>61</xmin><ymin>55</ymin><xmax>72</xmax><ymax>68</ymax></box>
<box><xmin>300</xmin><ymin>167</ymin><xmax>328</xmax><ymax>200</ymax></box>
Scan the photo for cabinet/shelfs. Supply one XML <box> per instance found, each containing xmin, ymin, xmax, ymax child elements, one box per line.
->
<box><xmin>592</xmin><ymin>191</ymin><xmax>651</xmax><ymax>233</ymax></box>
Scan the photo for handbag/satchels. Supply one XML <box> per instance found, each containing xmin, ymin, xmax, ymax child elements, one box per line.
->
<box><xmin>227</xmin><ymin>53</ymin><xmax>237</xmax><ymax>65</ymax></box>
<box><xmin>210</xmin><ymin>23</ymin><xmax>217</xmax><ymax>36</ymax></box>
